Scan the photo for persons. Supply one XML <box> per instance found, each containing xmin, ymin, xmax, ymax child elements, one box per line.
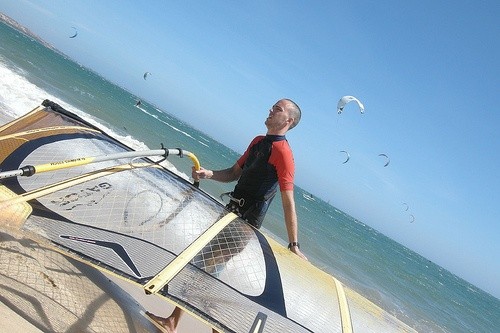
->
<box><xmin>145</xmin><ymin>97</ymin><xmax>306</xmax><ymax>333</ymax></box>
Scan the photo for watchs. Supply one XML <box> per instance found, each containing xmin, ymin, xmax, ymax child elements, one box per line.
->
<box><xmin>287</xmin><ymin>241</ymin><xmax>301</xmax><ymax>249</ymax></box>
